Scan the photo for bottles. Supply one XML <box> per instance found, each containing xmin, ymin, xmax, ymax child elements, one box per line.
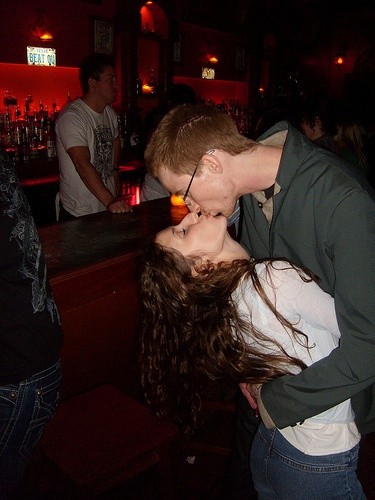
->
<box><xmin>31</xmin><ymin>99</ymin><xmax>59</xmax><ymax>159</ymax></box>
<box><xmin>117</xmin><ymin>112</ymin><xmax>129</xmax><ymax>136</ymax></box>
<box><xmin>231</xmin><ymin>101</ymin><xmax>252</xmax><ymax>136</ymax></box>
<box><xmin>218</xmin><ymin>100</ymin><xmax>231</xmax><ymax>115</ymax></box>
<box><xmin>0</xmin><ymin>95</ymin><xmax>30</xmax><ymax>162</ymax></box>
<box><xmin>136</xmin><ymin>79</ymin><xmax>140</xmax><ymax>94</ymax></box>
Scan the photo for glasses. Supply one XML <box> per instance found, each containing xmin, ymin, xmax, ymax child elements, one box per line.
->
<box><xmin>181</xmin><ymin>149</ymin><xmax>214</xmax><ymax>206</ymax></box>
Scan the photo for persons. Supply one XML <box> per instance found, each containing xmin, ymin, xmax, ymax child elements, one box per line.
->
<box><xmin>0</xmin><ymin>147</ymin><xmax>65</xmax><ymax>500</ymax></box>
<box><xmin>141</xmin><ymin>83</ymin><xmax>198</xmax><ymax>201</ymax></box>
<box><xmin>56</xmin><ymin>53</ymin><xmax>139</xmax><ymax>219</ymax></box>
<box><xmin>145</xmin><ymin>213</ymin><xmax>364</xmax><ymax>499</ymax></box>
<box><xmin>296</xmin><ymin>100</ymin><xmax>371</xmax><ymax>178</ymax></box>
<box><xmin>144</xmin><ymin>102</ymin><xmax>374</xmax><ymax>500</ymax></box>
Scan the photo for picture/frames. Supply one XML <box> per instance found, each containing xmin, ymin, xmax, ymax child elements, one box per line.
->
<box><xmin>233</xmin><ymin>46</ymin><xmax>248</xmax><ymax>74</ymax></box>
<box><xmin>88</xmin><ymin>15</ymin><xmax>114</xmax><ymax>59</ymax></box>
<box><xmin>171</xmin><ymin>32</ymin><xmax>184</xmax><ymax>66</ymax></box>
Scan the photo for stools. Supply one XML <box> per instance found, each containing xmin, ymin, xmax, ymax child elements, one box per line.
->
<box><xmin>37</xmin><ymin>383</ymin><xmax>178</xmax><ymax>500</ymax></box>
